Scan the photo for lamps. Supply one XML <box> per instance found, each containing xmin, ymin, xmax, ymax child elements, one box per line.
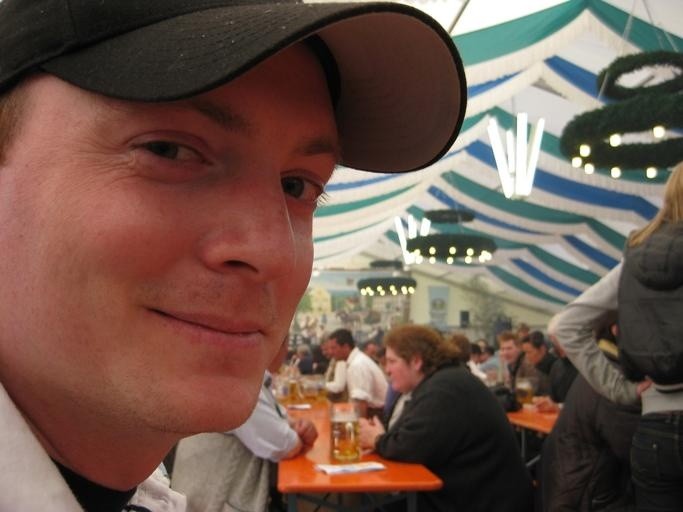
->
<box><xmin>356</xmin><ymin>95</ymin><xmax>546</xmax><ymax>299</ymax></box>
<box><xmin>555</xmin><ymin>0</ymin><xmax>682</xmax><ymax>181</ymax></box>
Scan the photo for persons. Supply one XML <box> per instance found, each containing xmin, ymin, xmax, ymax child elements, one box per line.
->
<box><xmin>547</xmin><ymin>162</ymin><xmax>683</xmax><ymax>512</ymax></box>
<box><xmin>0</xmin><ymin>2</ymin><xmax>470</xmax><ymax>511</ymax></box>
<box><xmin>170</xmin><ymin>323</ymin><xmax>641</xmax><ymax>510</ymax></box>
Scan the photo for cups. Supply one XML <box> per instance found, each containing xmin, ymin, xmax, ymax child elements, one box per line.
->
<box><xmin>515</xmin><ymin>377</ymin><xmax>534</xmax><ymax>404</ymax></box>
<box><xmin>329</xmin><ymin>405</ymin><xmax>361</xmax><ymax>463</ymax></box>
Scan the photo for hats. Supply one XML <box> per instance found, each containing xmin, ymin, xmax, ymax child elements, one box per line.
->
<box><xmin>1</xmin><ymin>0</ymin><xmax>469</xmax><ymax>175</ymax></box>
